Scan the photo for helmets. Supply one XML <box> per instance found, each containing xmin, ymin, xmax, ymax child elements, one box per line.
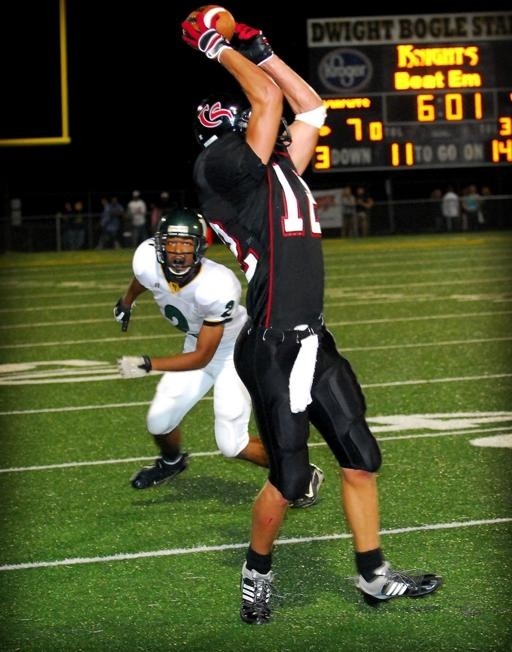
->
<box><xmin>193</xmin><ymin>80</ymin><xmax>292</xmax><ymax>147</ymax></box>
<box><xmin>155</xmin><ymin>208</ymin><xmax>207</xmax><ymax>269</ymax></box>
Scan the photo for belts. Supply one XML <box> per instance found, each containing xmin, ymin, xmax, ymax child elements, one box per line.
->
<box><xmin>247</xmin><ymin>312</ymin><xmax>325</xmax><ymax>344</ymax></box>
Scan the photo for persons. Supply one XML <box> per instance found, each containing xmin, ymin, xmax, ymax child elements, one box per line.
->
<box><xmin>112</xmin><ymin>210</ymin><xmax>326</xmax><ymax>508</ymax></box>
<box><xmin>65</xmin><ymin>189</ymin><xmax>173</xmax><ymax>250</ymax></box>
<box><xmin>354</xmin><ymin>186</ymin><xmax>374</xmax><ymax>236</ymax></box>
<box><xmin>338</xmin><ymin>186</ymin><xmax>358</xmax><ymax>239</ymax></box>
<box><xmin>180</xmin><ymin>11</ymin><xmax>441</xmax><ymax>625</ymax></box>
<box><xmin>431</xmin><ymin>185</ymin><xmax>490</xmax><ymax>231</ymax></box>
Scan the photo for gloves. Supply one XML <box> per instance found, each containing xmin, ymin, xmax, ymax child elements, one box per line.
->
<box><xmin>182</xmin><ymin>6</ymin><xmax>273</xmax><ymax>66</ymax></box>
<box><xmin>113</xmin><ymin>296</ymin><xmax>131</xmax><ymax>333</ymax></box>
<box><xmin>118</xmin><ymin>355</ymin><xmax>151</xmax><ymax>374</ymax></box>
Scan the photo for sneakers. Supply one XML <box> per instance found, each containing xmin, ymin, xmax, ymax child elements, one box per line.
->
<box><xmin>288</xmin><ymin>463</ymin><xmax>324</xmax><ymax>509</ymax></box>
<box><xmin>132</xmin><ymin>452</ymin><xmax>188</xmax><ymax>489</ymax></box>
<box><xmin>354</xmin><ymin>561</ymin><xmax>442</xmax><ymax>606</ymax></box>
<box><xmin>240</xmin><ymin>561</ymin><xmax>273</xmax><ymax>625</ymax></box>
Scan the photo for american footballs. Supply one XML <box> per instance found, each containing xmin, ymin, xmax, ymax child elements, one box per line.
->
<box><xmin>183</xmin><ymin>5</ymin><xmax>237</xmax><ymax>43</ymax></box>
